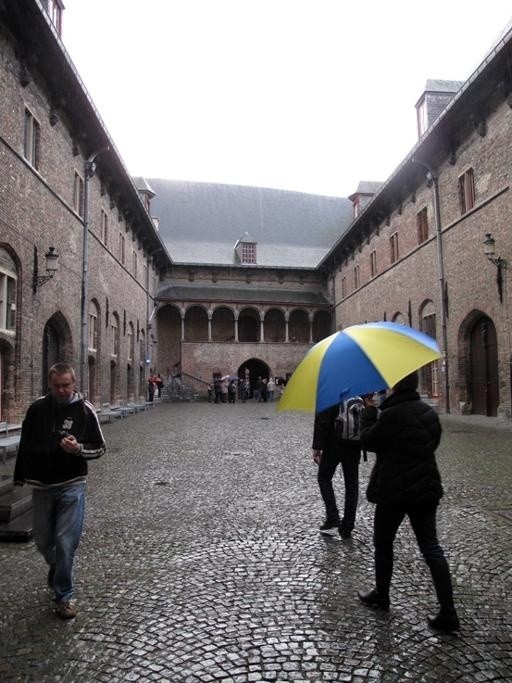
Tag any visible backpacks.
[334,395,367,441]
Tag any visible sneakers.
[320,515,343,530]
[60,596,76,620]
[337,527,352,538]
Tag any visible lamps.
[36,246,59,288]
[483,233,502,267]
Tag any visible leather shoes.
[358,589,390,610]
[427,608,460,631]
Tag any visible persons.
[208,371,289,405]
[313,396,367,537]
[11,361,106,620]
[147,373,156,405]
[155,373,163,397]
[360,366,460,631]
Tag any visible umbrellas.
[273,322,446,414]
